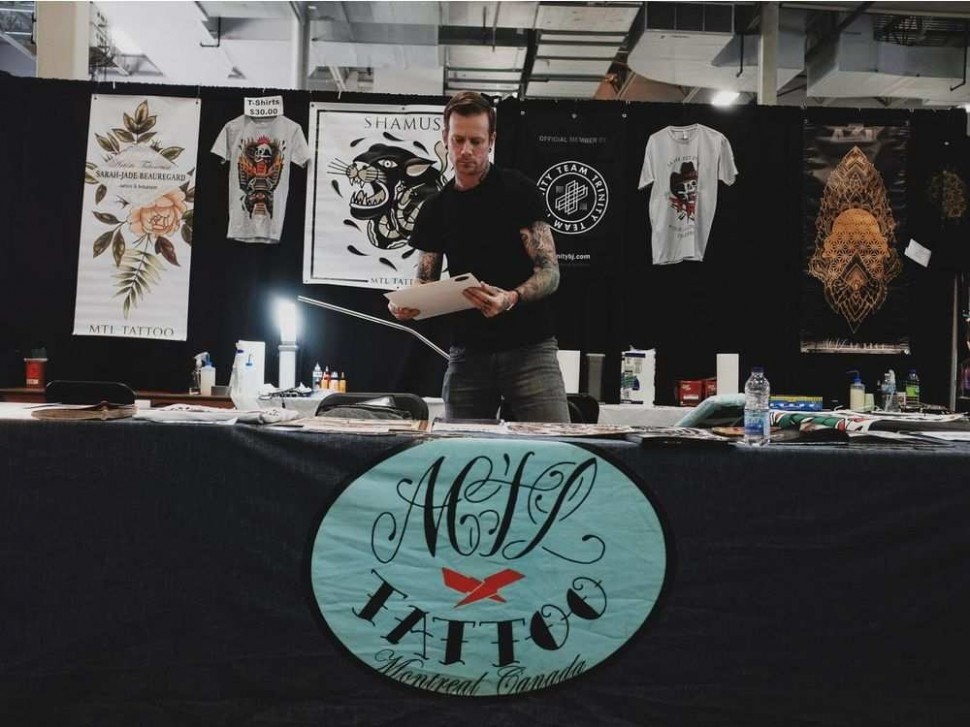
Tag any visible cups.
[23,358,48,388]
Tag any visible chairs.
[45,379,138,407]
[499,394,599,424]
[315,391,429,422]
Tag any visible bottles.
[201,354,216,394]
[881,373,896,413]
[744,368,770,447]
[904,369,919,408]
[312,362,346,394]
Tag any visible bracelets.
[512,289,522,303]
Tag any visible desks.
[0,400,970,727]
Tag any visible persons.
[388,92,571,423]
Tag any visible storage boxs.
[673,378,717,408]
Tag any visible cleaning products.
[846,370,865,412]
[191,350,220,399]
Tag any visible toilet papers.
[716,353,740,395]
